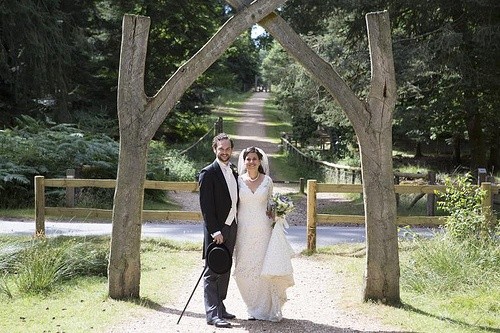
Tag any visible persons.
[232,146,295,322]
[199,134,241,328]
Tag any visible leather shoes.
[207,318,231,328]
[219,312,235,319]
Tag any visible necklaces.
[247,172,259,181]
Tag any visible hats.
[206,241,232,274]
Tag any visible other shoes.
[248,317,256,320]
